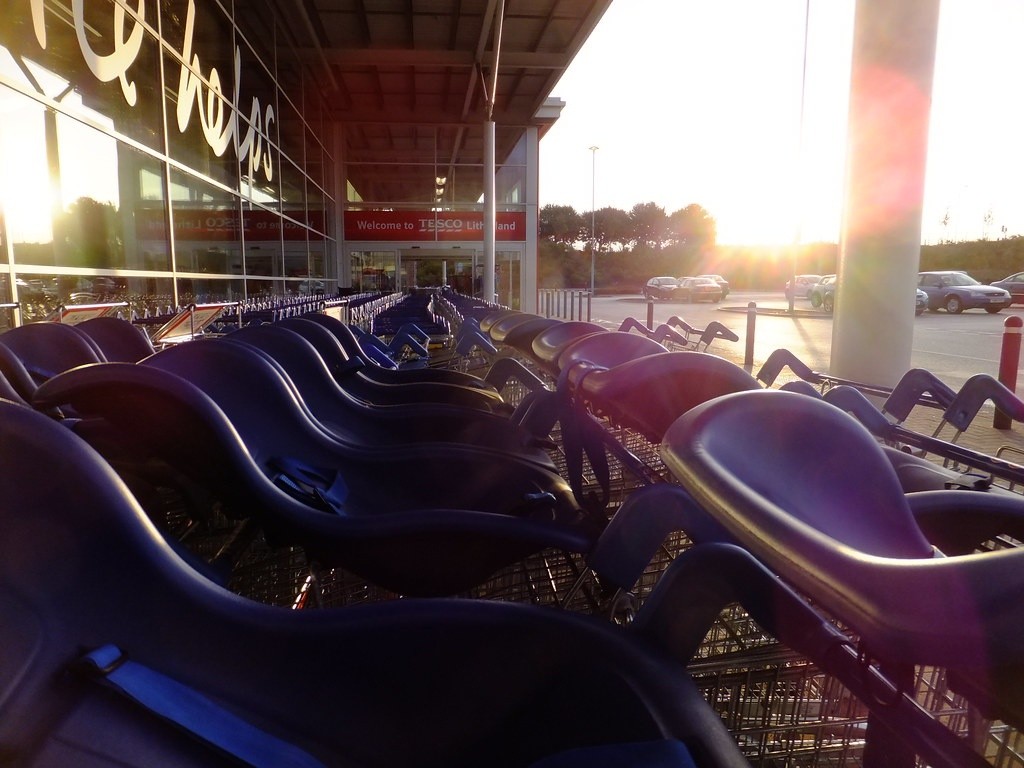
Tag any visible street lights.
[588,146,601,295]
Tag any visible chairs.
[0,284,1024,768]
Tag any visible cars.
[783,274,823,298]
[641,276,678,301]
[810,274,837,307]
[821,276,929,315]
[916,271,1012,315]
[670,276,723,303]
[990,271,1024,305]
[680,274,731,300]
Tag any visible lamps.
[433,175,446,203]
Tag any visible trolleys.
[0,283,1024,768]
